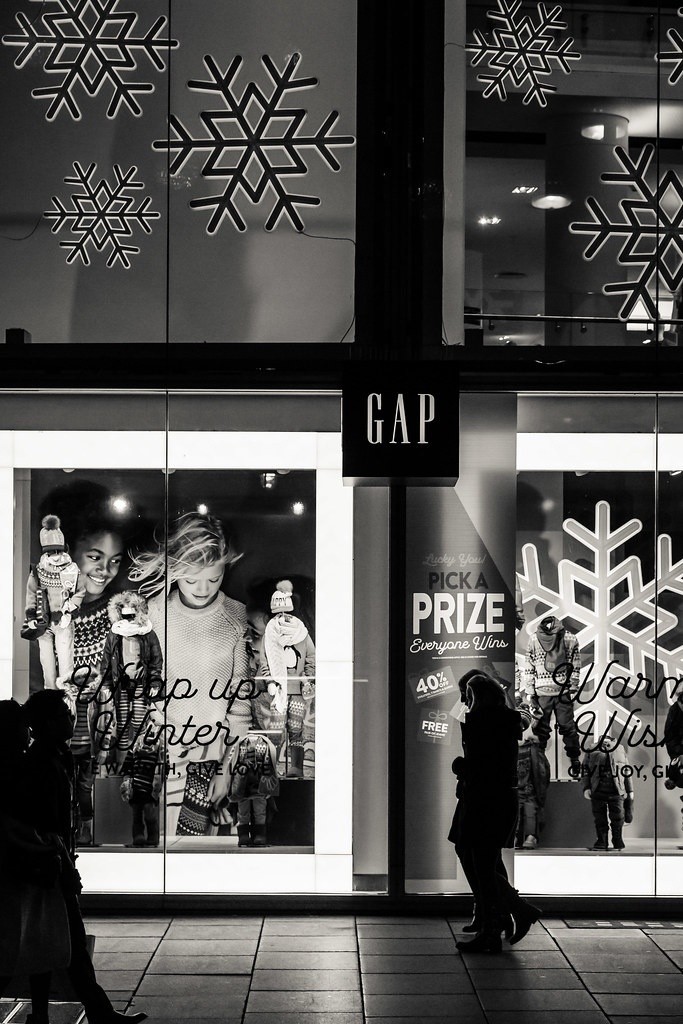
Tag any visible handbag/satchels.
[119,779,132,801]
[22,563,50,641]
[0,935,96,1001]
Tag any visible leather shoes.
[96,1009,148,1024]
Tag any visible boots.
[463,902,488,931]
[456,922,503,954]
[76,820,92,844]
[509,897,542,946]
[254,824,267,845]
[286,744,304,778]
[237,823,252,846]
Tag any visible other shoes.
[523,835,537,848]
[612,836,625,850]
[594,841,608,852]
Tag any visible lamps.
[261,471,278,488]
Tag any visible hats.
[40,515,64,552]
[270,581,293,613]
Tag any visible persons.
[242,574,315,779]
[101,591,163,763]
[25,515,86,690]
[123,721,170,845]
[48,479,146,668]
[581,735,634,850]
[447,669,542,952]
[132,512,252,836]
[260,580,315,776]
[515,702,547,848]
[525,615,583,779]
[0,689,148,1024]
[228,734,279,846]
